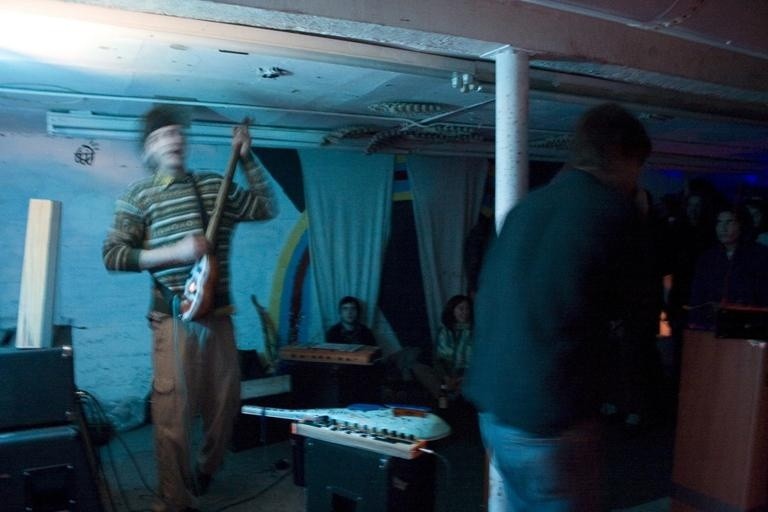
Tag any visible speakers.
[0,425,106,512]
[302,438,438,512]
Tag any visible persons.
[323,295,377,407]
[462,161,554,290]
[458,101,664,512]
[411,294,476,400]
[103,105,280,512]
[629,184,767,343]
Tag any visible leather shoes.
[190,466,211,494]
